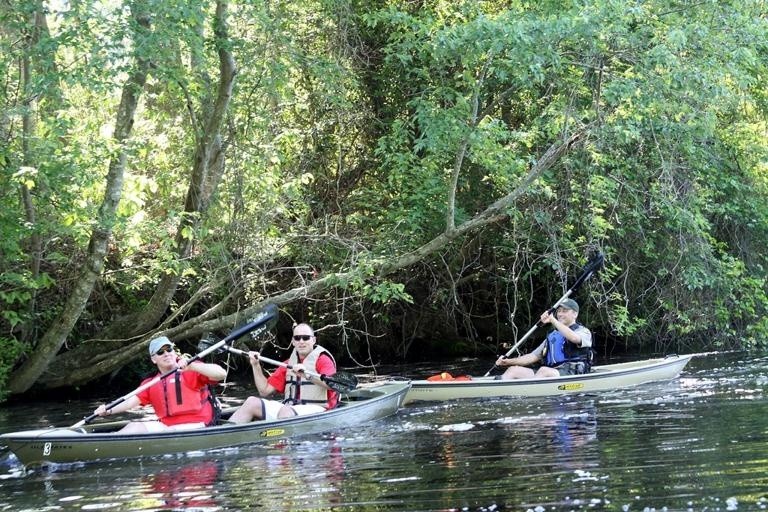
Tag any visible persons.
[496,298,593,380]
[223,324,342,427]
[93,336,228,435]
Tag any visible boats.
[403,354,695,405]
[0,378,413,472]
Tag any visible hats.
[149,336,175,356]
[559,299,580,314]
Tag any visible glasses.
[292,335,315,341]
[156,347,172,355]
